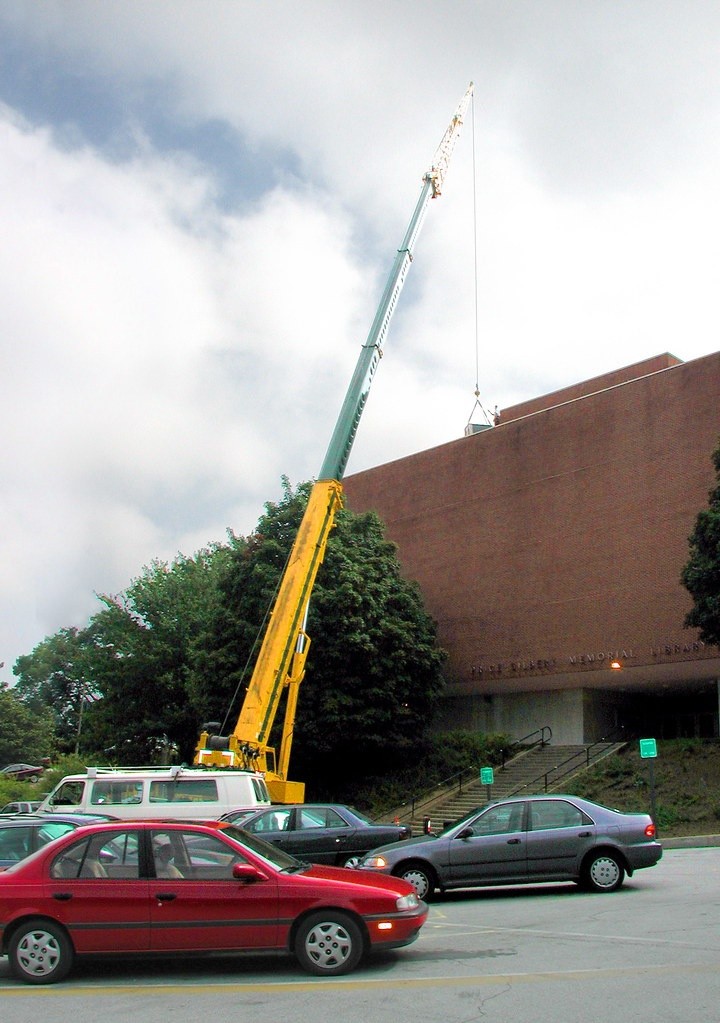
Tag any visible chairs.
[54,842,109,878]
[159,844,185,878]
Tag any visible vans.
[33,766,274,844]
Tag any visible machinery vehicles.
[106,79,480,805]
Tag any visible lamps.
[610,662,621,669]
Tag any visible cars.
[160,803,414,866]
[0,763,45,783]
[0,818,430,985]
[0,801,218,872]
[355,794,665,903]
